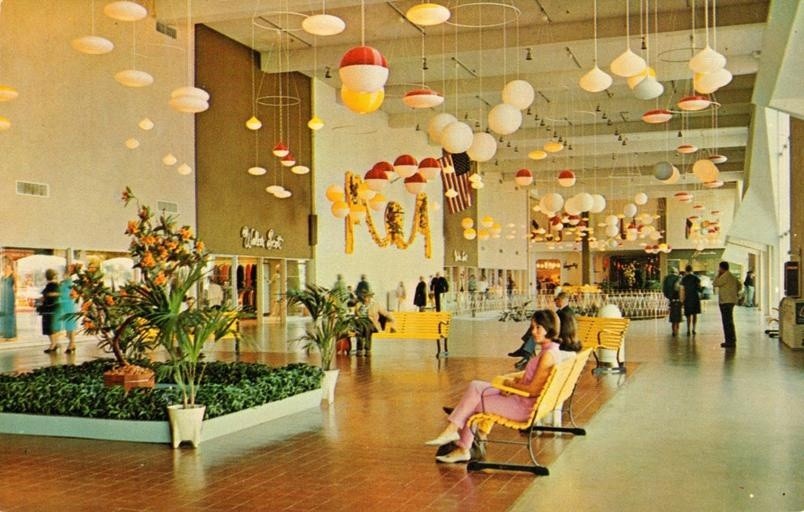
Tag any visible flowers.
[60,182,208,374]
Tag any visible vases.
[98,363,157,397]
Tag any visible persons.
[38,269,62,353]
[662,262,756,347]
[59,271,79,352]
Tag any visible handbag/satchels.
[679,284,686,303]
[435,416,488,463]
[744,281,748,287]
[559,342,582,352]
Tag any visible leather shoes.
[435,447,473,462]
[721,340,736,348]
[424,431,461,446]
[507,348,530,359]
[515,358,528,368]
[442,406,455,415]
[687,330,695,336]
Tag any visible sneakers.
[356,349,370,357]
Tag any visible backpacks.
[663,274,679,299]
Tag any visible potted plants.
[106,242,255,453]
[285,310,381,404]
[276,278,359,356]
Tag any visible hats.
[670,267,678,273]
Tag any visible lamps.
[580,1,731,242]
[325,171,387,222]
[246,0,345,199]
[468,173,485,189]
[445,189,458,198]
[177,163,192,176]
[645,245,659,254]
[444,166,455,174]
[0,116,11,130]
[0,84,18,103]
[505,223,526,240]
[364,0,534,190]
[170,2,210,114]
[162,153,177,166]
[529,215,660,247]
[338,1,389,114]
[72,2,154,87]
[125,137,140,149]
[515,21,605,215]
[461,209,502,241]
[606,136,648,218]
[139,118,154,131]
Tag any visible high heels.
[64,347,76,353]
[44,344,58,353]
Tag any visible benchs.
[465,344,596,475]
[569,314,633,376]
[333,305,453,362]
